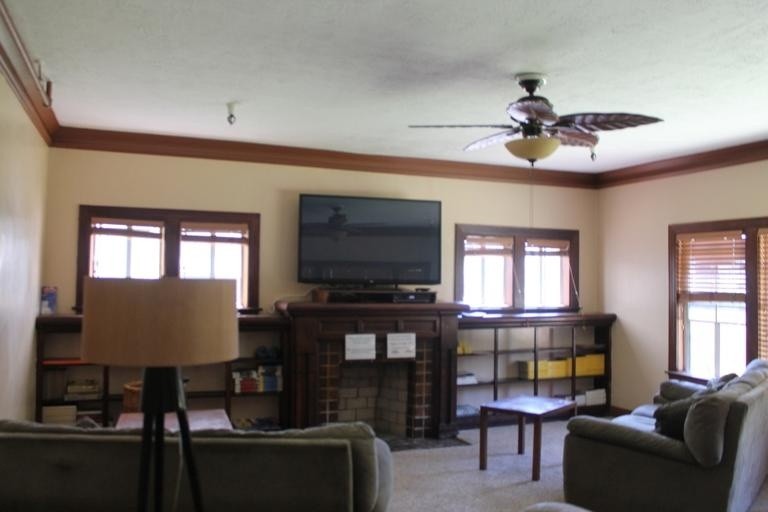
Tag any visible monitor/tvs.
[297,194,441,285]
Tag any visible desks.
[480,393,578,482]
[114,409,233,431]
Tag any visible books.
[231,364,284,393]
[42,377,104,429]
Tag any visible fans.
[407,74,663,151]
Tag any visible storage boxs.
[517,354,607,380]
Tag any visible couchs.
[563,358,767,511]
[0,416,391,512]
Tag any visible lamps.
[81,277,238,510]
[505,121,559,165]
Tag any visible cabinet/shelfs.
[224,318,294,431]
[457,313,616,431]
[35,317,109,427]
